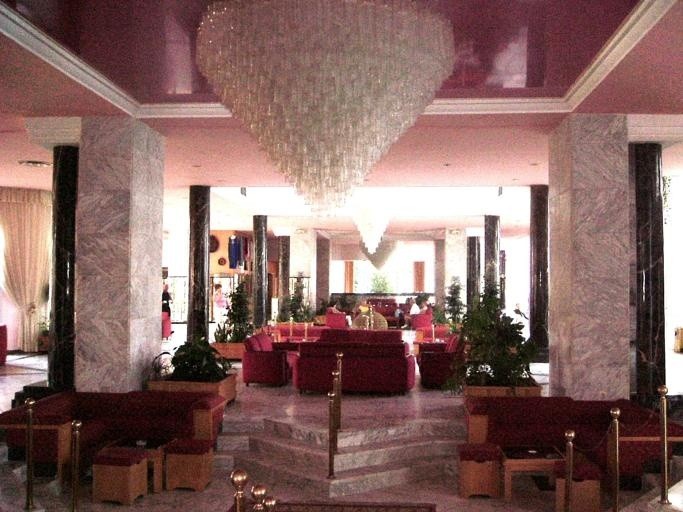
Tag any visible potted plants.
[147,334,238,405]
[440,284,542,414]
[211,281,254,359]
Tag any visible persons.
[326,300,349,327]
[160,283,172,340]
[400,301,428,330]
[214,283,227,320]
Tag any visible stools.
[92,448,148,505]
[458,443,500,498]
[164,439,213,492]
[553,461,601,512]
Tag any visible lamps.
[197,0,454,222]
[358,238,395,270]
[352,214,388,254]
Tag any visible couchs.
[464,395,683,488]
[243,300,464,394]
[0,390,226,490]
